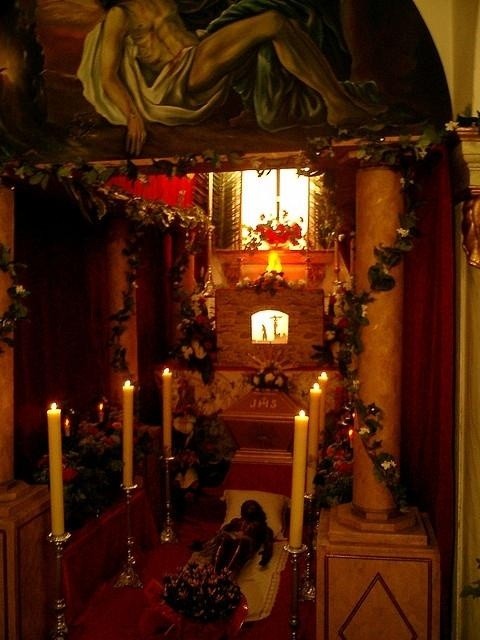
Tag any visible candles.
[47,373,170,538]
[290,376,327,549]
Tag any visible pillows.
[219,490,289,541]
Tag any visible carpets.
[196,535,289,622]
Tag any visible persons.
[190,499,274,579]
